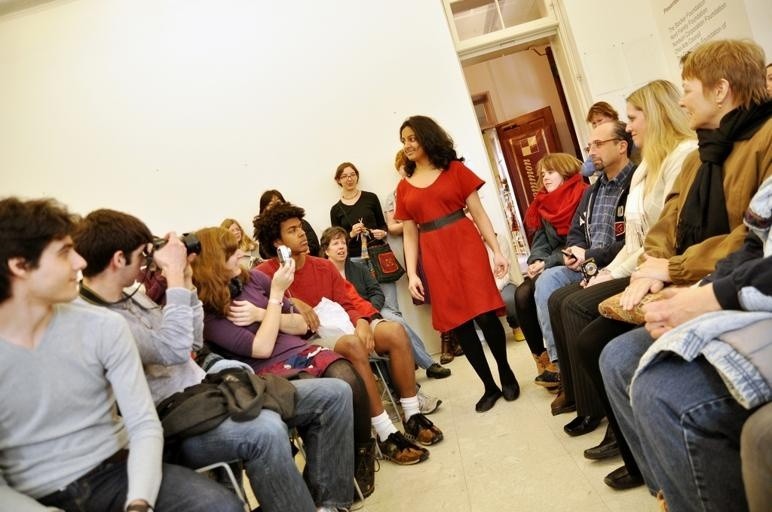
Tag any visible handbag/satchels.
[597,285,687,328]
[362,228,405,285]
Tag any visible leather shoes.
[551,381,575,416]
[583,424,620,460]
[602,465,645,490]
[512,325,528,342]
[472,384,501,413]
[564,412,605,436]
[453,340,465,356]
[439,348,454,364]
[501,372,521,402]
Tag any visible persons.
[508,39,771,510]
[1,162,453,510]
[386,117,521,412]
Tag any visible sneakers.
[376,429,430,467]
[426,361,452,379]
[416,385,442,415]
[402,412,443,446]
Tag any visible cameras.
[581,257,599,284]
[149,234,203,258]
[277,245,292,263]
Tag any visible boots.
[532,347,561,393]
[352,438,377,499]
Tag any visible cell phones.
[562,249,577,261]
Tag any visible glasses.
[340,173,357,180]
[586,136,623,151]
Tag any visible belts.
[418,207,466,233]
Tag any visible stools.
[293,427,364,511]
[195,458,250,512]
[370,360,401,421]
